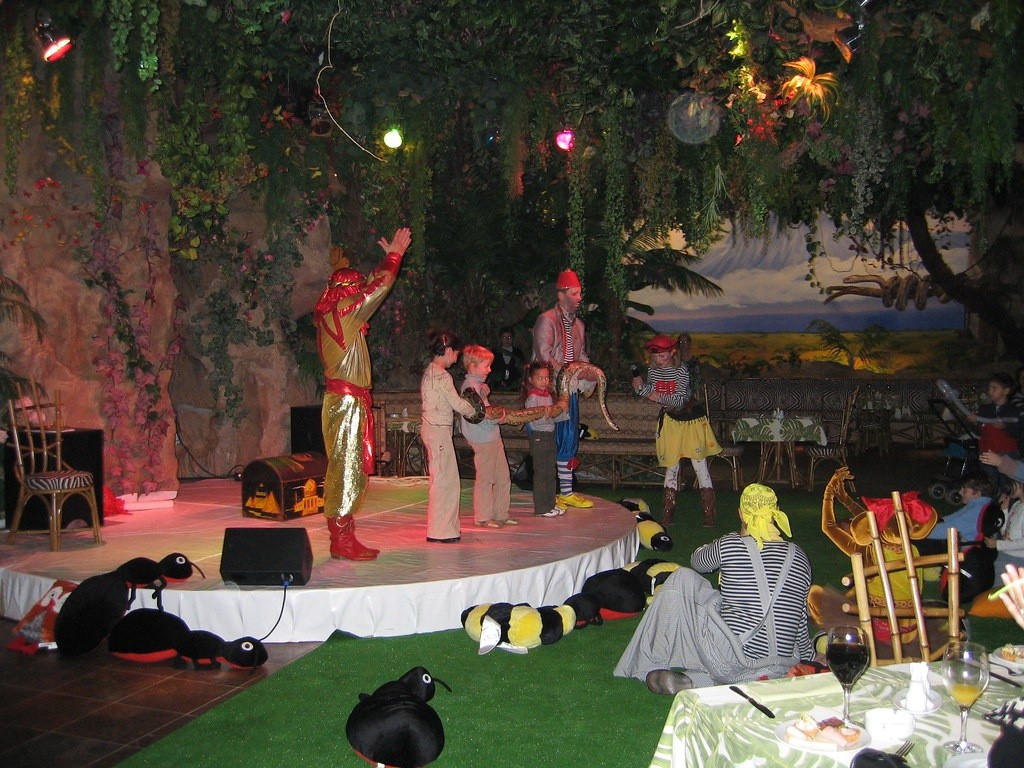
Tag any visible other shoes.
[536,509,559,518]
[553,506,565,515]
[427,536,460,544]
[646,669,693,696]
[503,516,517,525]
[475,521,499,529]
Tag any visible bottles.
[402,408,409,418]
[773,410,784,418]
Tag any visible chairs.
[849,490,968,670]
[693,382,744,490]
[806,386,862,495]
[7,375,102,554]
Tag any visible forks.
[895,740,915,758]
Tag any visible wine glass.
[943,642,989,753]
[826,626,870,729]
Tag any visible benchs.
[372,390,687,491]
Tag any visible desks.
[731,414,828,489]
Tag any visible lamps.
[34,18,73,63]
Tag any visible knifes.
[729,685,775,720]
[989,670,1021,688]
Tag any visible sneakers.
[558,494,594,507]
[554,502,567,510]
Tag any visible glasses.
[502,336,512,339]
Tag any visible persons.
[313,226,411,559]
[525,363,567,517]
[420,332,465,543]
[632,333,723,528]
[532,269,597,508]
[613,483,827,694]
[927,367,1024,630]
[491,327,525,388]
[461,344,522,529]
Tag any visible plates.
[943,752,988,768]
[774,721,871,754]
[892,688,942,715]
[993,645,1024,666]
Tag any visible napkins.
[905,662,934,711]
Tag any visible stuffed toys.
[940,488,1011,606]
[56,552,292,669]
[346,665,453,768]
[619,497,674,553]
[808,466,948,660]
[461,557,685,654]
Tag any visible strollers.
[926,398,981,508]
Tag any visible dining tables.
[649,656,1024,768]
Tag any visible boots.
[661,486,675,529]
[327,514,377,561]
[699,487,715,528]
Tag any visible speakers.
[220,528,314,586]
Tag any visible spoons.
[987,660,1022,676]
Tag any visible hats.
[645,335,678,354]
[557,268,581,290]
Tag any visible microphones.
[630,365,642,392]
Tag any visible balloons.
[936,379,1018,423]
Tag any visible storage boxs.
[241,451,328,522]
